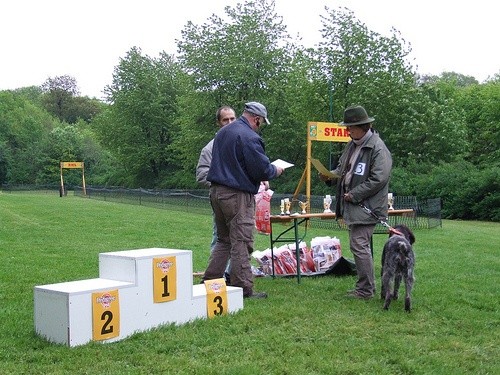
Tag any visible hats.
[337,105,375,126]
[245,102,270,125]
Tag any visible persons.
[201,102,283,299]
[196,107,237,278]
[318,105,393,299]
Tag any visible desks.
[269,208,413,283]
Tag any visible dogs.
[380,225,416,312]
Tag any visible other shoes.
[345,294,374,300]
[243,291,267,298]
[346,289,375,296]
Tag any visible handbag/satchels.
[254,181,274,236]
[252,236,341,274]
